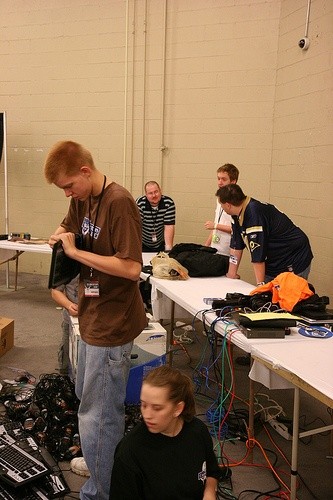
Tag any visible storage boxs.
[0,316,14,357]
[68,313,166,405]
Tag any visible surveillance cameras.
[298,37,310,50]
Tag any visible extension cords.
[176,320,194,331]
[267,414,296,439]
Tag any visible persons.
[215,184,314,297]
[108,364,221,500]
[204,163,239,273]
[135,180,176,317]
[42,139,149,500]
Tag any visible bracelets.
[214,223,217,229]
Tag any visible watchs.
[256,281,266,288]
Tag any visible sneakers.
[70,457,90,477]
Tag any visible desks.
[0,238,53,291]
[140,253,333,500]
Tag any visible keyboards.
[0,421,70,500]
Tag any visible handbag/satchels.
[48,233,83,289]
[150,251,189,280]
[168,242,229,277]
